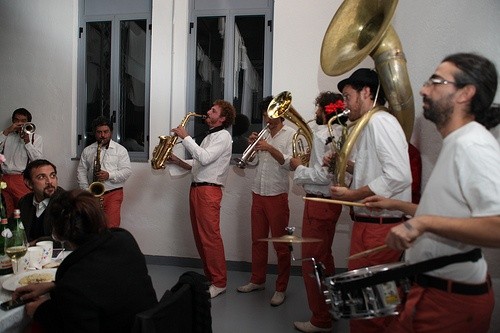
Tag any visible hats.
[338,68,387,104]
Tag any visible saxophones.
[88,140,108,199]
[150,109,208,170]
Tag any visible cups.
[11,241,53,274]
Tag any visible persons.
[8,159,67,249]
[13,188,158,333]
[363,53,500,333]
[322,68,413,333]
[236,96,297,305]
[76,117,131,228]
[0,107,43,217]
[166,100,236,297]
[289,91,356,333]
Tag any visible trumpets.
[17,123,36,138]
[233,121,273,170]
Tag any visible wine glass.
[4,230,27,274]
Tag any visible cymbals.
[256,233,327,246]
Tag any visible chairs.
[127,271,213,333]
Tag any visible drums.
[320,261,413,322]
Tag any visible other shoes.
[271,291,285,306]
[209,285,226,298]
[293,321,332,333]
[237,282,265,293]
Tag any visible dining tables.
[0,240,71,333]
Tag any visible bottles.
[0,191,28,275]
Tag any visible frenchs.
[319,0,415,189]
[266,89,315,167]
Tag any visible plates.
[2,268,57,291]
[43,258,64,268]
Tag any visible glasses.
[430,75,461,84]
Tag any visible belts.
[104,186,123,195]
[191,182,220,187]
[355,215,404,223]
[414,275,492,295]
